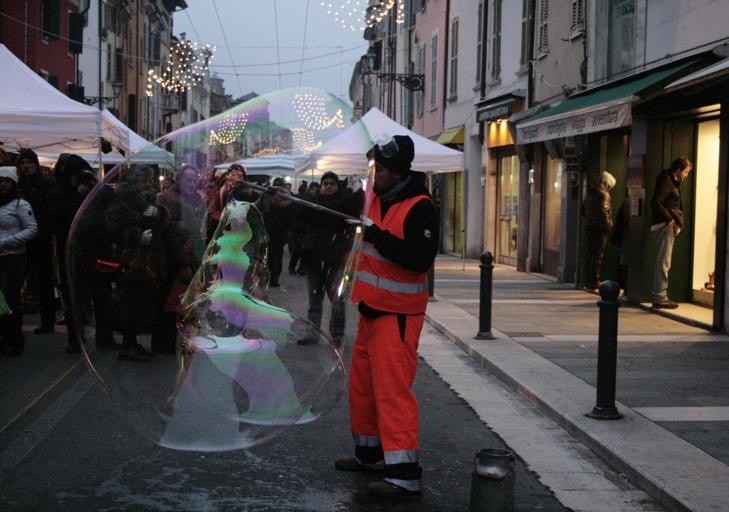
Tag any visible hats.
[0,166,19,185]
[366,135,415,174]
[321,171,339,186]
[17,147,38,166]
[126,163,155,186]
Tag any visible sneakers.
[368,480,423,498]
[297,332,321,346]
[33,323,56,334]
[653,300,678,309]
[335,455,377,473]
[66,341,84,354]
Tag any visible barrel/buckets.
[471,448,514,512]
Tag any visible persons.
[581,169,618,294]
[609,197,628,298]
[331,135,442,503]
[2,148,208,361]
[647,155,694,311]
[203,149,365,346]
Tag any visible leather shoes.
[118,344,155,363]
